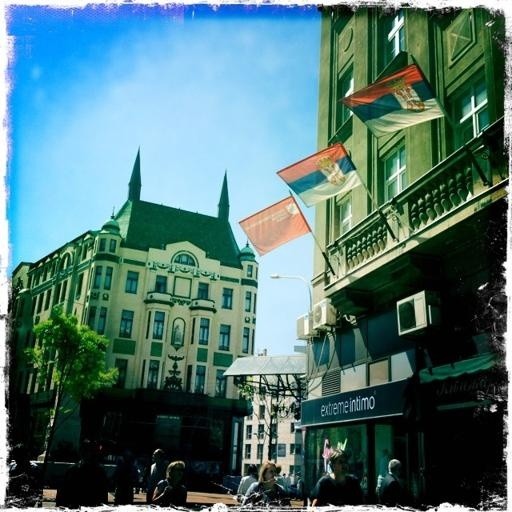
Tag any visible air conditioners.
[295,289,443,340]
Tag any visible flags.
[274,139,361,212]
[335,63,445,139]
[237,196,311,261]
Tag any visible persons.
[108,448,187,507]
[8,443,41,506]
[54,440,108,506]
[238,461,303,511]
[378,459,406,505]
[310,449,364,508]
